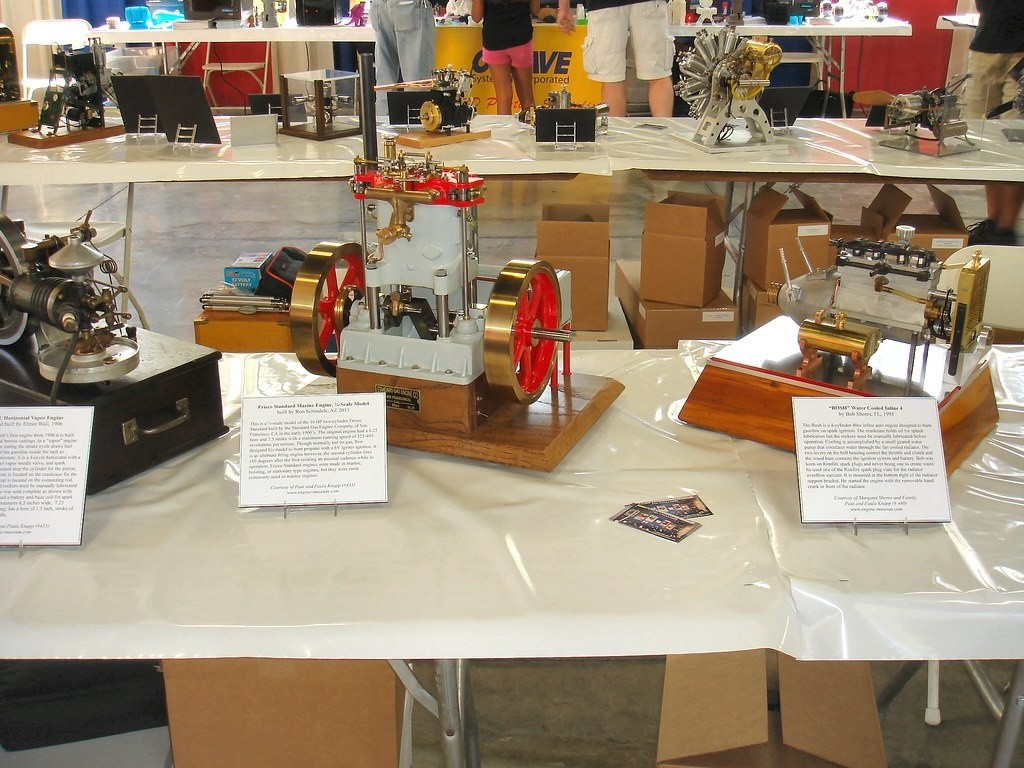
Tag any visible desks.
[91,16,377,93]
[668,12,914,112]
[2,317,1024,768]
[430,9,608,112]
[0,113,1024,329]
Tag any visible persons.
[555,0,678,117]
[369,0,437,117]
[470,0,543,114]
[958,1,1024,235]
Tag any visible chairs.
[201,41,271,108]
[19,19,96,99]
